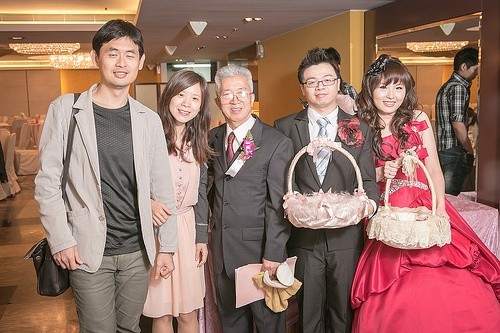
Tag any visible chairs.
[420,103,435,127]
[0,112,47,197]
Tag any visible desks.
[196,193,500,333]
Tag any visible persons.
[273,50,379,333]
[349,54,500,333]
[141,69,218,333]
[323,46,359,102]
[205,64,296,333]
[436,47,479,198]
[35,18,178,333]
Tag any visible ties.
[226,133,235,162]
[313,117,331,182]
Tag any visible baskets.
[282,139,368,228]
[366,153,452,249]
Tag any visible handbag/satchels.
[24,236,71,297]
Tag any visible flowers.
[338,117,364,149]
[239,130,261,162]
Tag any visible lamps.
[8,42,80,56]
[165,46,177,56]
[407,42,471,52]
[187,20,207,38]
[49,53,98,69]
[440,22,455,37]
[147,64,156,70]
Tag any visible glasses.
[302,76,339,88]
[220,90,251,104]
